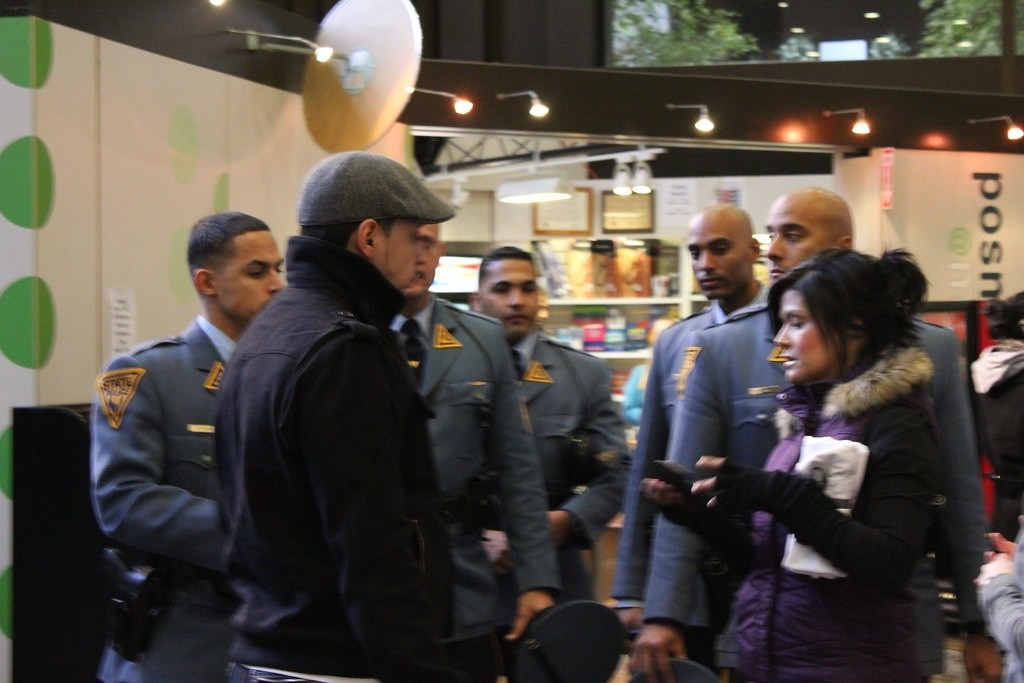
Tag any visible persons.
[621,316,676,424]
[972,511,1024,683]
[214,151,458,683]
[391,223,562,683]
[89,210,287,683]
[971,292,1024,542]
[627,187,1002,683]
[641,247,948,683]
[470,246,631,630]
[610,202,764,657]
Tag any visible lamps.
[495,149,572,203]
[450,175,471,209]
[613,152,653,196]
[414,89,1024,139]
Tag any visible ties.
[399,319,427,383]
[511,349,524,381]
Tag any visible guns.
[102,546,158,664]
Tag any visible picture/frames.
[533,187,594,236]
[602,189,654,234]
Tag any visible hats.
[507,600,623,683]
[296,151,457,225]
[628,659,722,683]
